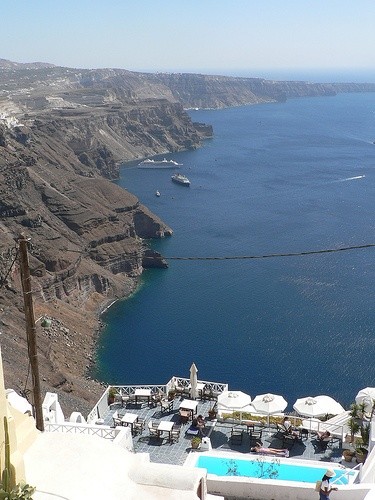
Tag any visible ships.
[136,157,180,169]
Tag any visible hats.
[324,469,336,478]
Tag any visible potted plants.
[109,387,116,404]
[355,437,368,464]
[343,448,354,462]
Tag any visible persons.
[192,414,208,437]
[283,417,299,439]
[251,442,283,455]
[311,430,331,442]
[319,469,338,500]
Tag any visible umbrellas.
[252,393,288,426]
[292,397,327,436]
[355,387,375,414]
[217,391,252,432]
[190,362,199,398]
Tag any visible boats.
[171,173,190,185]
[156,190,160,196]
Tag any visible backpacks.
[315,480,322,492]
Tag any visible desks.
[112,383,341,449]
